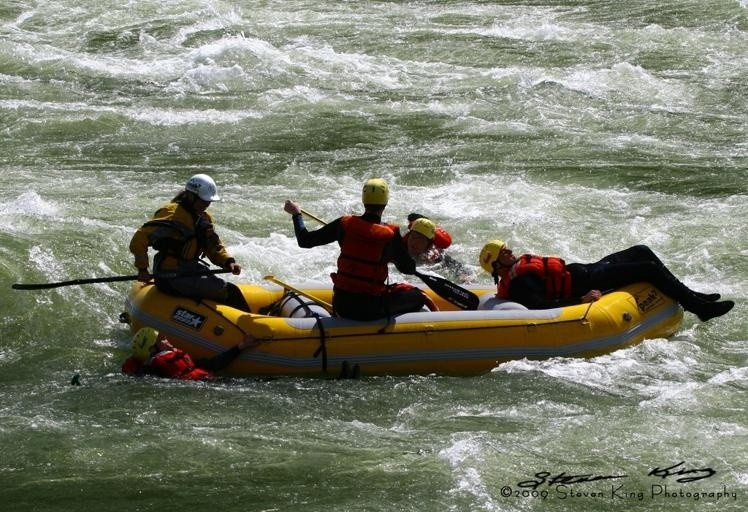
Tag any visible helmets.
[131,327,159,361]
[478,240,505,273]
[409,218,435,240]
[185,174,219,201]
[362,179,389,205]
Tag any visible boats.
[119,279,686,375]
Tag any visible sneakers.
[695,292,733,322]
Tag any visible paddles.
[13,269,231,289]
[299,208,480,313]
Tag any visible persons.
[284,178,425,323]
[121,327,260,385]
[479,240,735,323]
[129,174,252,314]
[401,213,463,274]
